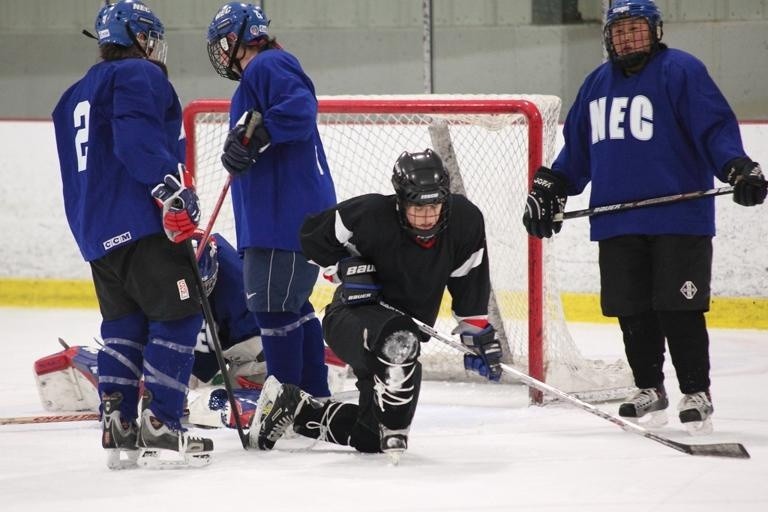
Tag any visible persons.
[206,0,338,402]
[521,0,767,433]
[63,227,268,428]
[246,148,502,464]
[52,0,216,468]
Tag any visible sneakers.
[619,388,668,417]
[378,422,409,450]
[679,391,713,423]
[257,383,310,450]
[138,407,214,454]
[102,410,139,450]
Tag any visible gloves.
[221,111,270,176]
[461,324,503,383]
[338,256,381,307]
[722,156,767,206]
[151,163,201,245]
[523,166,567,239]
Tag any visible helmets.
[190,227,220,304]
[207,3,269,81]
[603,0,663,75]
[95,0,168,64]
[391,148,450,239]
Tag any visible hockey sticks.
[185,238,250,451]
[379,301,750,459]
